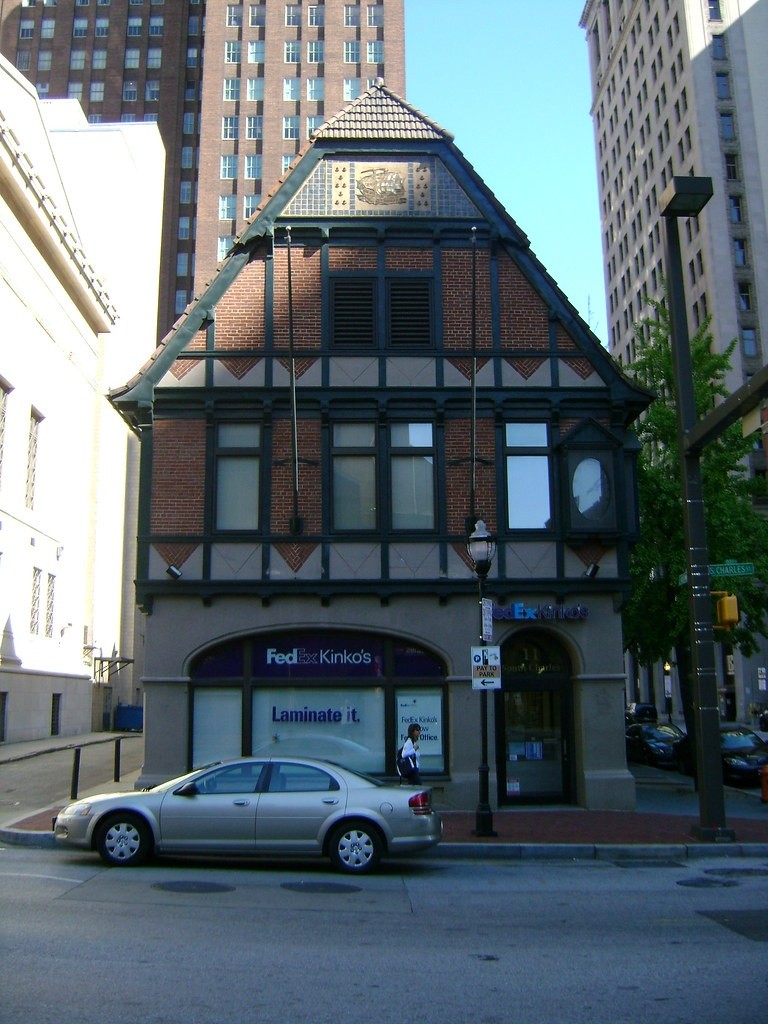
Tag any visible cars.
[671,723,768,788]
[230,733,381,774]
[626,702,671,724]
[52,752,442,875]
[625,721,688,769]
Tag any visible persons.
[400,723,422,783]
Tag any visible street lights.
[656,173,738,845]
[465,519,499,839]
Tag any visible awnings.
[95,656,135,675]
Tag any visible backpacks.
[395,736,410,782]
